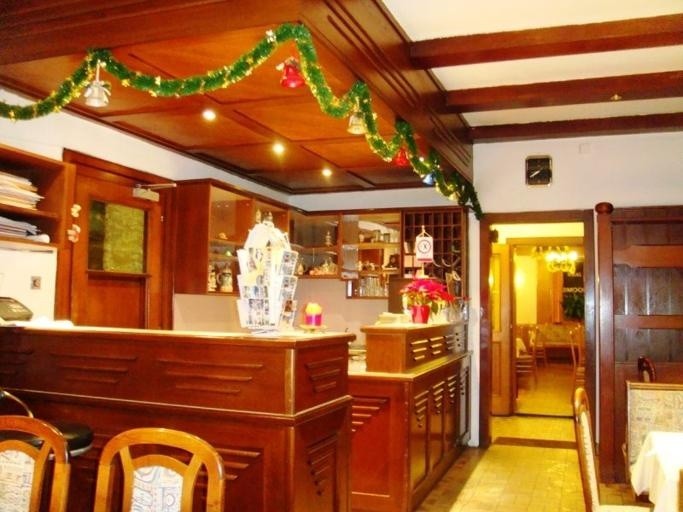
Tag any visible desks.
[645,432,683,512]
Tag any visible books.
[0,162,50,246]
[234,219,300,334]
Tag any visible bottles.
[325,229,332,246]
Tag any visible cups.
[208,264,233,292]
[359,230,390,243]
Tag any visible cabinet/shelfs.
[0,144,77,252]
[174,177,470,300]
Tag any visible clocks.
[525,155,553,189]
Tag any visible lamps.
[545,245,577,273]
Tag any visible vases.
[410,303,431,323]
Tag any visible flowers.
[400,279,454,305]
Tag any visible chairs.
[0,415,227,512]
[572,387,651,512]
[515,323,586,391]
